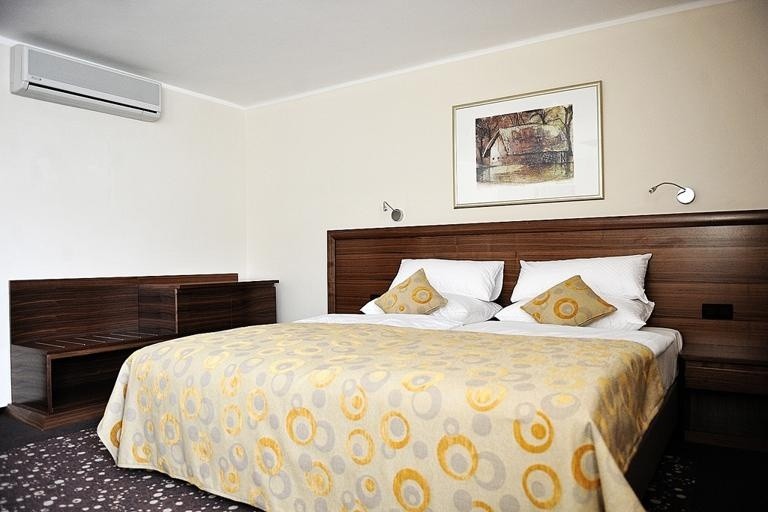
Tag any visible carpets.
[0,428,697,511]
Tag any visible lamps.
[382,200,405,223]
[648,181,696,204]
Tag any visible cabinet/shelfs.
[6,273,279,431]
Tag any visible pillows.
[360,252,656,332]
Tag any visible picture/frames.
[450,79,605,208]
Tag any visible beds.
[98,311,683,512]
